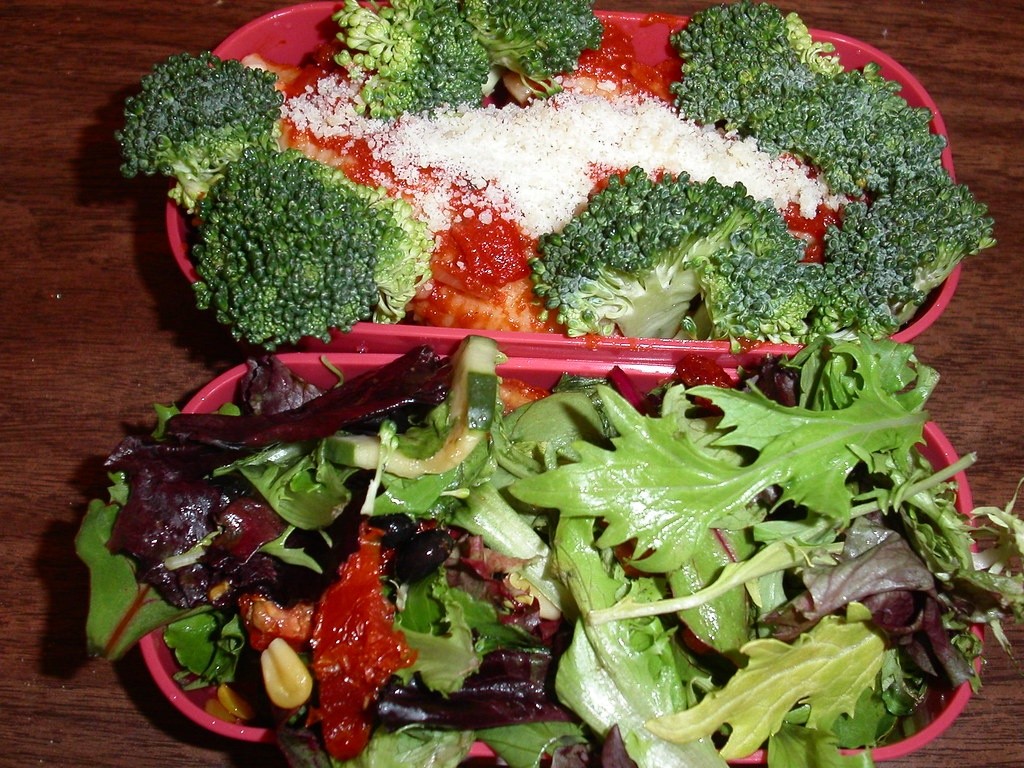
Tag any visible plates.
[166,0,963,365]
[136,353,984,761]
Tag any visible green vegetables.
[72,335,1024,768]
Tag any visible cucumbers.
[324,334,500,480]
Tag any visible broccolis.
[116,0,997,352]
